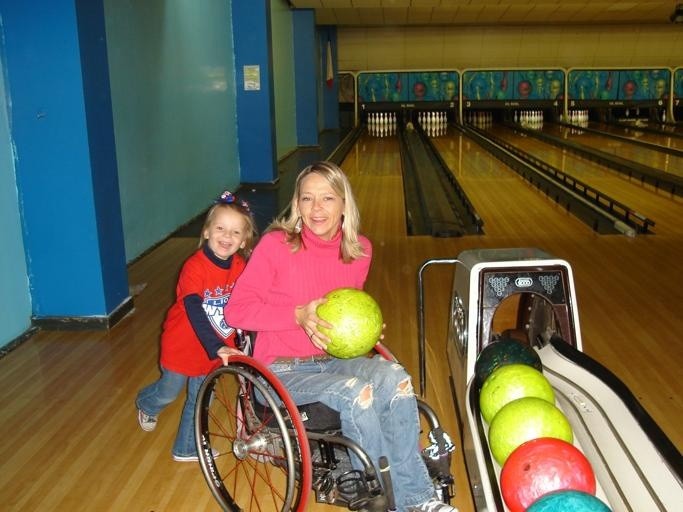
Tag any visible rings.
[311,333,314,338]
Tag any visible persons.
[223,161,458,512]
[136,190,259,462]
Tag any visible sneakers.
[406,497,459,512]
[174,449,219,462]
[139,409,158,432]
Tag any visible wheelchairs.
[194,329,455,512]
